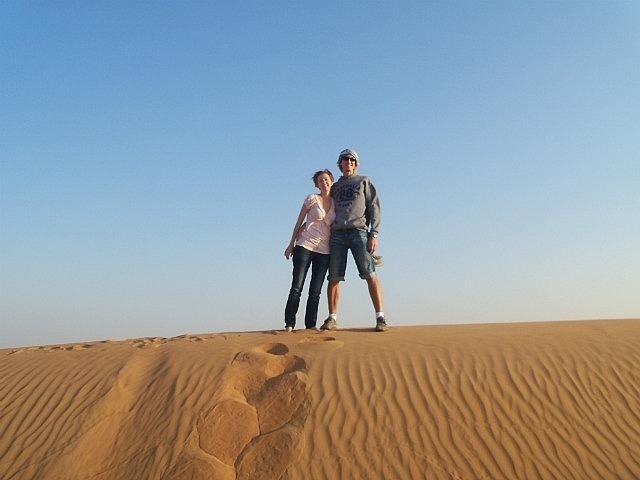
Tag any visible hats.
[338,149,358,172]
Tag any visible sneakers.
[376,316,387,331]
[320,317,336,330]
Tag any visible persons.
[285,169,338,333]
[295,147,390,331]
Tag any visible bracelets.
[369,234,380,237]
